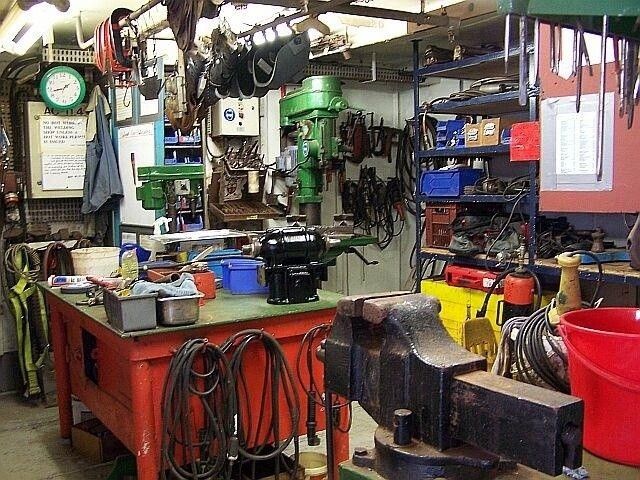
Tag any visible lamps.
[0,1,72,56]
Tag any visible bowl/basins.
[70,246,121,278]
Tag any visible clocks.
[39,65,85,108]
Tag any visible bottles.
[120,244,139,280]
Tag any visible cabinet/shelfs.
[529,19,640,278]
[410,10,538,272]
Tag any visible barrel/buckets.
[70,246,121,277]
[556,306,640,469]
[289,449,327,479]
